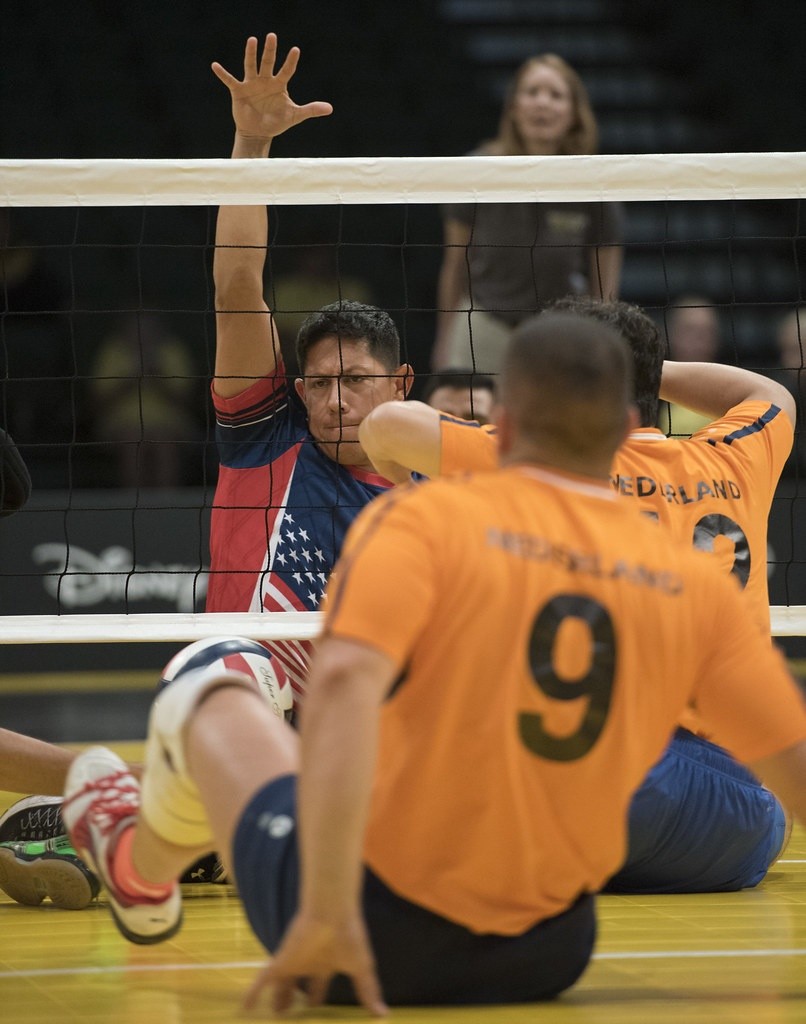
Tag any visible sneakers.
[0,834,101,910]
[62,744,185,946]
[0,795,67,841]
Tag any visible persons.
[211,33,806,734]
[0,240,806,1017]
